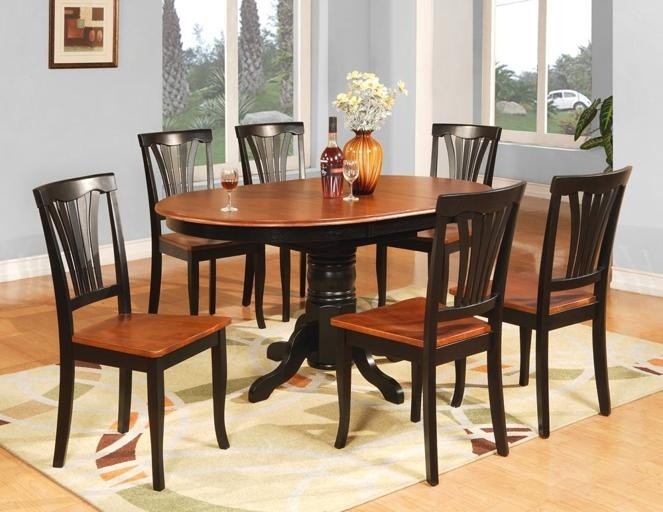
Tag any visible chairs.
[330,181,528,486]
[235,122,306,322]
[449,165,633,439]
[137,128,267,329]
[33,172,230,491]
[376,123,502,306]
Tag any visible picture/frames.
[49,0,119,69]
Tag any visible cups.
[342,159,360,201]
[220,167,239,212]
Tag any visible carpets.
[1,281,663,512]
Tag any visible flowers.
[332,71,408,131]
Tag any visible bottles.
[320,117,343,199]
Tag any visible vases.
[343,129,383,195]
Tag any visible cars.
[546,89,590,110]
